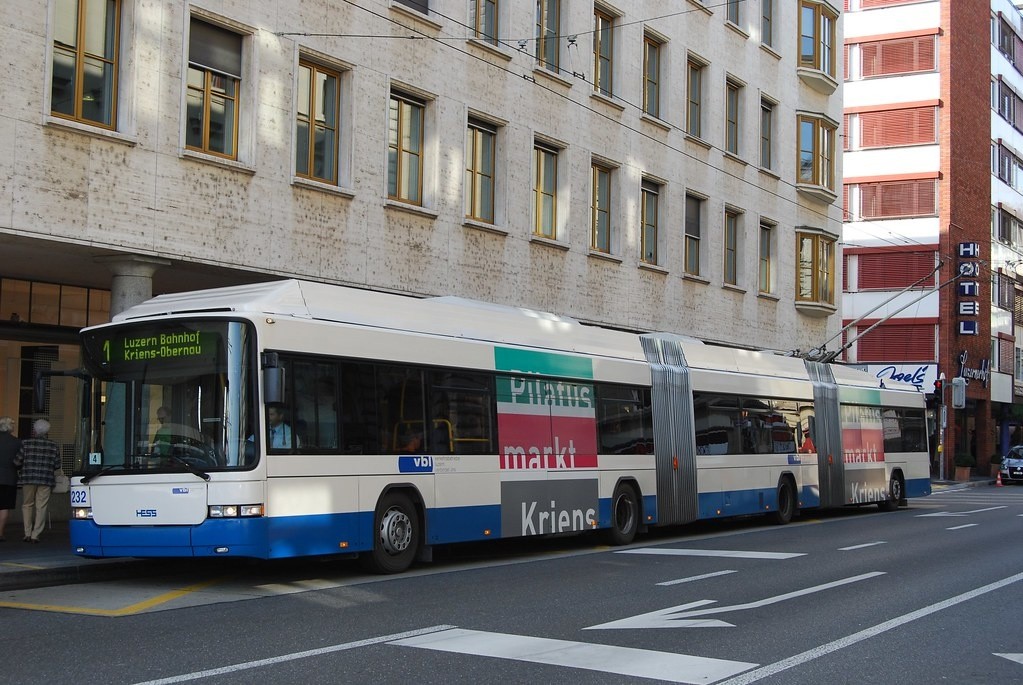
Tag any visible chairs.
[296,419,309,450]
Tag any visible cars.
[999,445,1023,484]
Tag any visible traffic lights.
[933,380,941,402]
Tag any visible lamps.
[1006,259,1023,268]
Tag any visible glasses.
[157,416,166,420]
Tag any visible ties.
[270,429,276,448]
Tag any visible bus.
[32,277,932,574]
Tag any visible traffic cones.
[995,470,1004,487]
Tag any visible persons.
[396,429,421,451]
[1010,425,1023,446]
[0,416,22,542]
[248,406,301,448]
[13,419,61,544]
[151,406,173,454]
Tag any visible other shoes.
[32,539,40,544]
[22,536,31,542]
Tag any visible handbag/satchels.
[50,476,71,494]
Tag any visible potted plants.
[953,452,975,482]
[990,453,1002,479]
